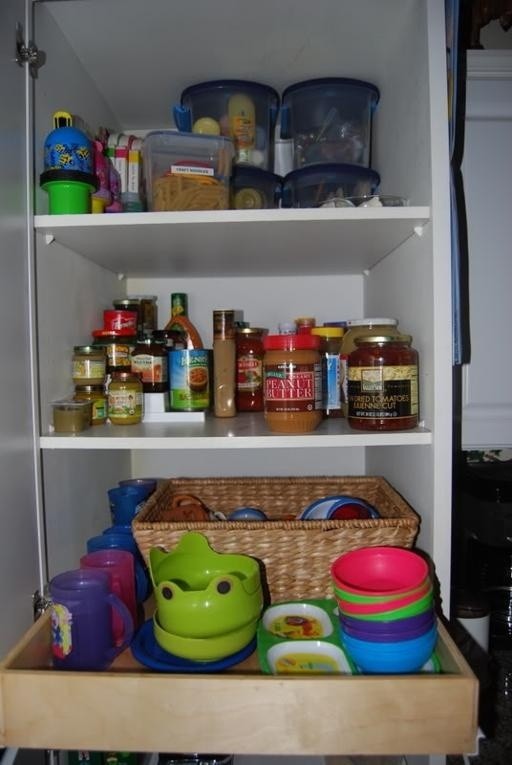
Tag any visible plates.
[128,619,256,672]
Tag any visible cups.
[47,569,133,671]
[120,479,158,496]
[86,536,145,607]
[107,486,149,523]
[80,549,137,646]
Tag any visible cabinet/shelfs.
[458,47,511,454]
[1,1,483,764]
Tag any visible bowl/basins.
[152,534,258,662]
[329,547,439,675]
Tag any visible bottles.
[311,327,345,417]
[164,292,205,350]
[263,335,324,433]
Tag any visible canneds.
[238,317,419,432]
[72,294,181,426]
[168,349,211,413]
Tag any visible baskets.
[132,476,420,606]
